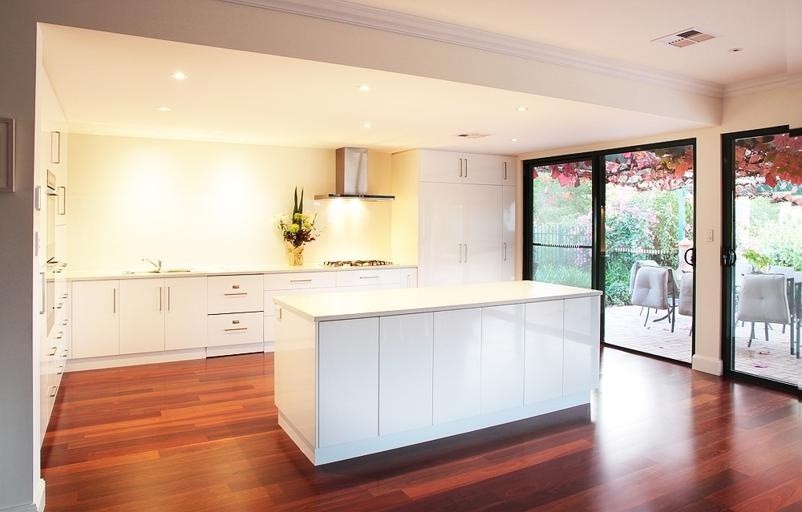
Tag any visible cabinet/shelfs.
[207,273,267,360]
[390,151,504,186]
[263,271,319,355]
[317,267,419,290]
[504,187,518,280]
[390,180,504,280]
[31,61,72,448]
[63,279,120,373]
[273,278,605,467]
[504,154,519,187]
[120,275,207,370]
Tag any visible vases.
[283,240,305,266]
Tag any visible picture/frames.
[1,117,17,196]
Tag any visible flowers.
[278,185,323,245]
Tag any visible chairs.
[629,255,802,362]
